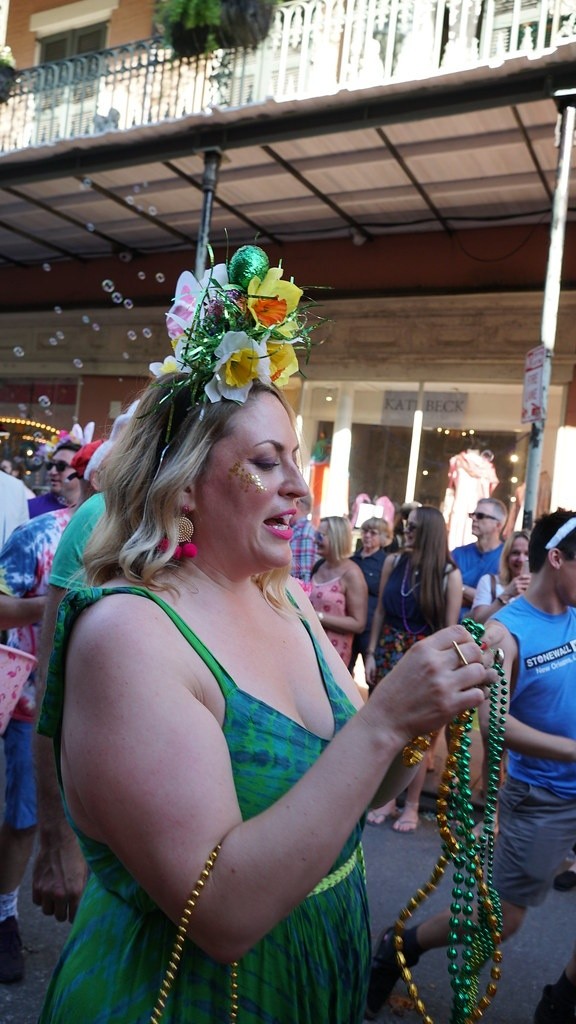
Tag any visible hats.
[69,439,109,480]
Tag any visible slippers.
[366,806,397,827]
[392,815,421,834]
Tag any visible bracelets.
[498,596,507,605]
[317,612,323,621]
[365,651,375,656]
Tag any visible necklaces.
[151,841,238,1024]
[401,560,420,597]
[392,619,507,1024]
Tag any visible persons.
[289,489,534,841]
[0,404,140,983]
[367,508,576,1024]
[37,374,497,1024]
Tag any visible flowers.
[136,244,336,443]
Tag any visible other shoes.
[363,927,420,1019]
[427,750,436,771]
[534,984,576,1024]
[553,870,576,892]
[471,820,498,849]
[0,916,27,987]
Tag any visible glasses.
[313,530,327,542]
[43,457,73,473]
[403,520,417,532]
[468,512,500,522]
[362,528,379,537]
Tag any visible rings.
[453,641,468,666]
